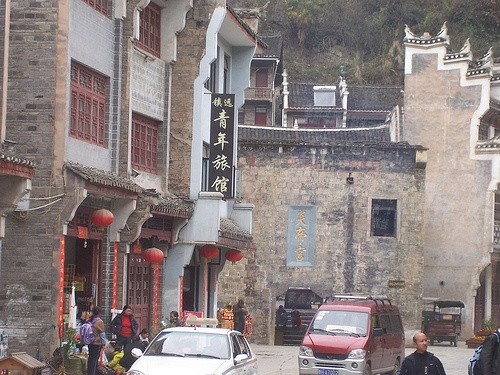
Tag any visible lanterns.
[225,249,243,265]
[201,244,219,262]
[91,208,113,231]
[143,247,165,270]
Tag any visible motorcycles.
[422,299,465,347]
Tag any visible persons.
[291,306,302,329]
[400,332,447,375]
[165,306,202,327]
[479,328,500,375]
[233,299,248,337]
[87,306,106,375]
[108,305,156,375]
[224,304,234,320]
[276,305,288,328]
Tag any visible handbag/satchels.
[97,364,115,375]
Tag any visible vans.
[298,292,405,375]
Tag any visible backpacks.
[468,330,500,375]
[80,317,101,345]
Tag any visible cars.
[125,326,258,375]
